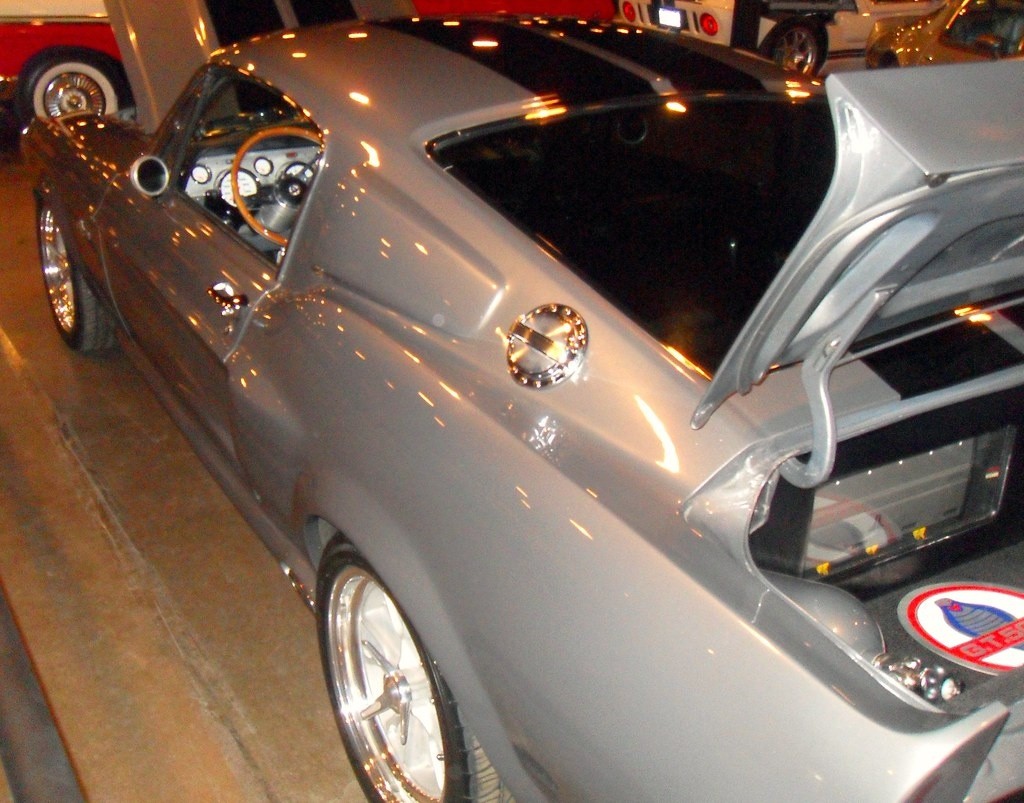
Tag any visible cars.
[21,16,1024,802]
[863,0,1024,71]
[0,1,616,130]
[617,0,948,76]
